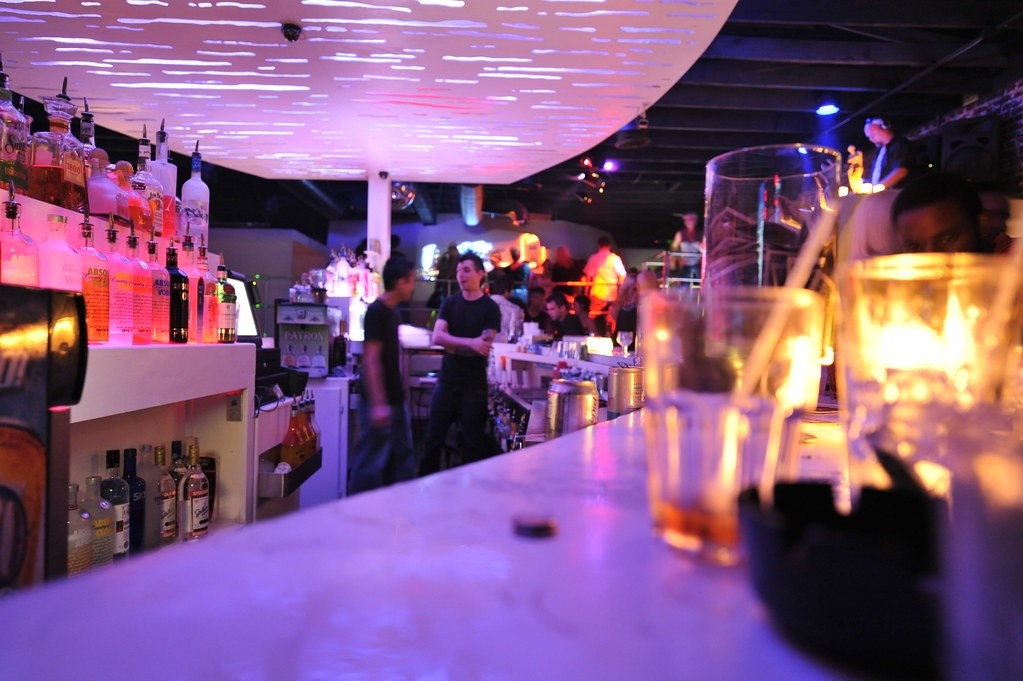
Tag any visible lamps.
[569,153,605,204]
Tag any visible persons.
[584,236,627,338]
[428,231,677,354]
[825,164,1023,257]
[417,249,503,478]
[856,113,919,189]
[347,254,418,494]
[672,212,702,287]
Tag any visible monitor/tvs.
[227,270,262,349]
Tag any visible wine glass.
[617,330,633,358]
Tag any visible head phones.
[865,117,891,129]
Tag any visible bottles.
[297,345,310,367]
[553,363,604,397]
[67,440,210,577]
[311,345,326,368]
[279,389,321,470]
[333,319,347,368]
[283,344,296,367]
[0,52,238,346]
[484,387,528,453]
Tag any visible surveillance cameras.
[280,21,304,42]
[379,171,389,179]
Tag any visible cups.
[698,143,840,507]
[347,267,380,298]
[313,287,326,305]
[557,340,577,360]
[638,283,826,569]
[835,186,1022,532]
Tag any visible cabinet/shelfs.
[68,344,359,576]
